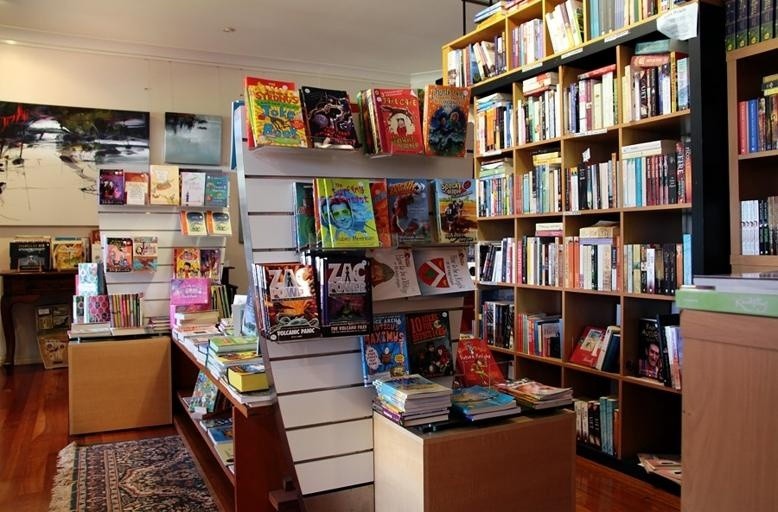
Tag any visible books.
[316,178,332,249]
[694,273,778,292]
[180,209,208,237]
[205,176,228,209]
[293,182,316,253]
[229,100,245,170]
[99,168,125,205]
[412,247,478,296]
[635,39,688,55]
[643,0,670,19]
[370,181,391,248]
[479,301,514,350]
[207,425,233,444]
[71,322,111,334]
[172,309,271,404]
[617,304,621,326]
[622,51,690,123]
[738,74,778,154]
[199,249,221,283]
[106,237,133,273]
[243,74,307,150]
[371,375,452,427]
[596,326,620,370]
[535,223,563,231]
[150,164,179,205]
[406,309,455,379]
[512,18,543,68]
[133,235,159,273]
[452,387,521,421]
[532,152,560,161]
[67,330,111,338]
[477,237,516,284]
[624,233,692,296]
[53,236,90,270]
[9,234,53,272]
[207,210,233,237]
[535,230,563,237]
[515,164,562,215]
[564,236,622,293]
[37,333,70,369]
[518,311,563,359]
[181,172,206,207]
[518,234,563,288]
[675,285,778,319]
[497,377,573,410]
[601,334,621,373]
[386,175,431,245]
[108,292,144,329]
[423,84,471,159]
[312,178,322,249]
[473,7,504,24]
[517,71,561,147]
[148,317,170,330]
[533,157,561,166]
[431,177,479,245]
[637,453,681,485]
[211,285,230,319]
[477,158,514,217]
[189,370,232,414]
[573,396,619,457]
[214,442,234,466]
[475,0,505,19]
[476,93,513,154]
[172,247,201,278]
[657,313,681,390]
[621,138,692,207]
[545,0,584,54]
[361,314,409,388]
[200,417,232,431]
[457,337,505,391]
[566,153,619,212]
[447,31,506,87]
[125,171,150,205]
[366,249,421,301]
[579,226,620,239]
[589,0,638,40]
[301,251,374,337]
[505,0,523,10]
[637,317,663,382]
[569,325,607,367]
[741,196,778,256]
[323,178,380,247]
[578,63,616,80]
[301,86,361,150]
[358,87,424,159]
[91,229,103,264]
[563,72,619,133]
[251,261,323,342]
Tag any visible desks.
[0,268,78,375]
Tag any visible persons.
[321,199,329,225]
[758,97,766,114]
[371,256,394,287]
[639,342,662,380]
[182,262,192,279]
[771,113,776,122]
[329,197,356,237]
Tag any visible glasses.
[332,208,350,219]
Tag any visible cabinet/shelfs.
[372,406,575,512]
[725,37,778,279]
[93,162,227,325]
[234,99,475,512]
[67,335,177,447]
[673,309,776,512]
[172,334,302,512]
[442,0,688,501]
